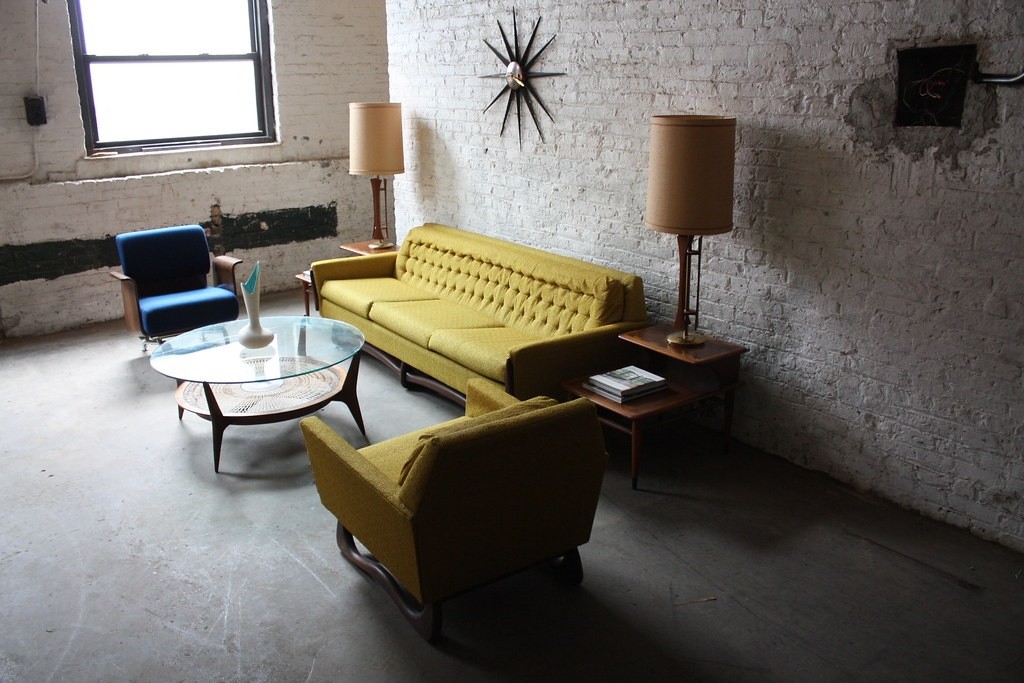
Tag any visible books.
[583,365,666,404]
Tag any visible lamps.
[348,101,405,250]
[645,114,735,346]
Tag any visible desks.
[294,238,401,316]
[555,322,747,489]
[150,316,366,473]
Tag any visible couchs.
[109,225,244,352]
[299,377,608,643]
[310,223,644,408]
